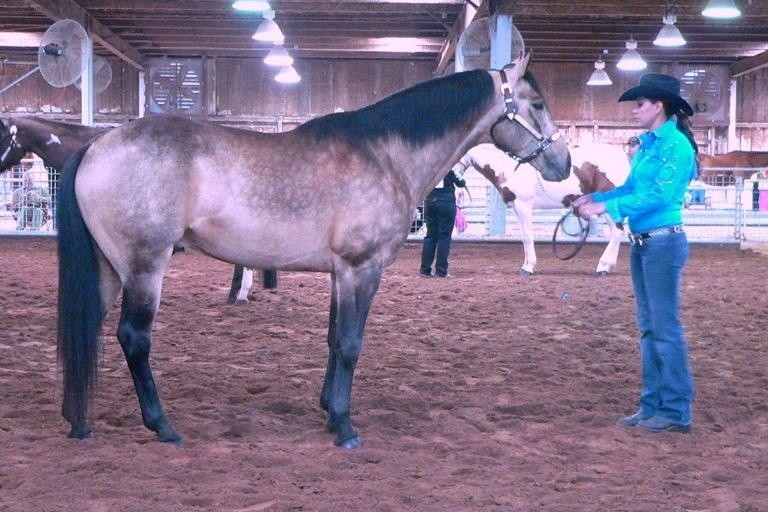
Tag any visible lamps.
[584,13,613,88]
[650,1,687,53]
[613,30,649,77]
[231,1,302,89]
[701,0,742,23]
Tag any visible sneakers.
[434,273,457,279]
[418,271,436,279]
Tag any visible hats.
[617,71,694,117]
[625,136,640,145]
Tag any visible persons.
[12,172,52,232]
[624,137,642,166]
[420,170,466,278]
[570,74,701,432]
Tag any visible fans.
[72,51,114,98]
[38,16,92,89]
[456,15,525,75]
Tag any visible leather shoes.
[638,415,693,434]
[616,407,656,428]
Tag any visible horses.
[451,142,633,276]
[1,114,278,306]
[696,150,768,187]
[54,47,572,452]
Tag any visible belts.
[626,225,685,242]
